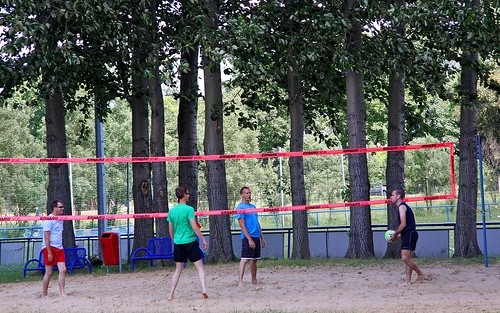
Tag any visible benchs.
[23,248,93,277]
[131,235,206,271]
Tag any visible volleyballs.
[384,230,400,243]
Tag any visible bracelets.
[395,231,399,235]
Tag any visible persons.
[167,186,209,301]
[388,188,426,287]
[236,187,266,288]
[43,199,68,300]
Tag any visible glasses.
[54,205,64,208]
[185,192,190,195]
